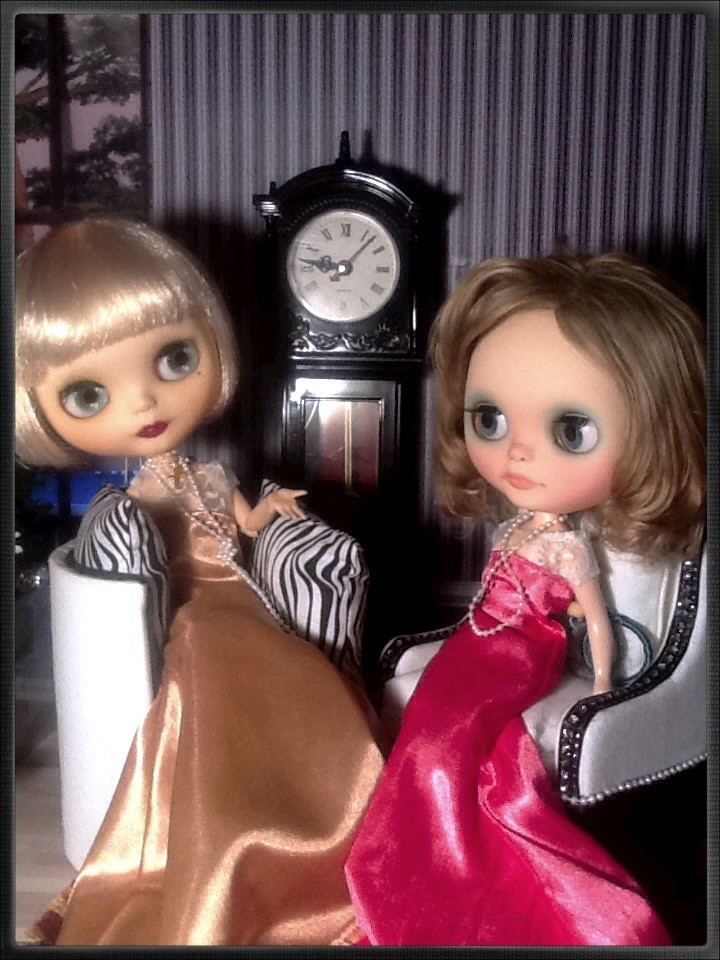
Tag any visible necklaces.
[469,511,566,639]
[143,451,297,639]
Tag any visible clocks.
[252,132,452,585]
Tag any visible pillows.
[248,479,369,682]
[73,485,178,661]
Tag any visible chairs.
[46,542,154,871]
[374,532,709,813]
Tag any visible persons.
[15,213,392,945]
[343,252,706,947]
